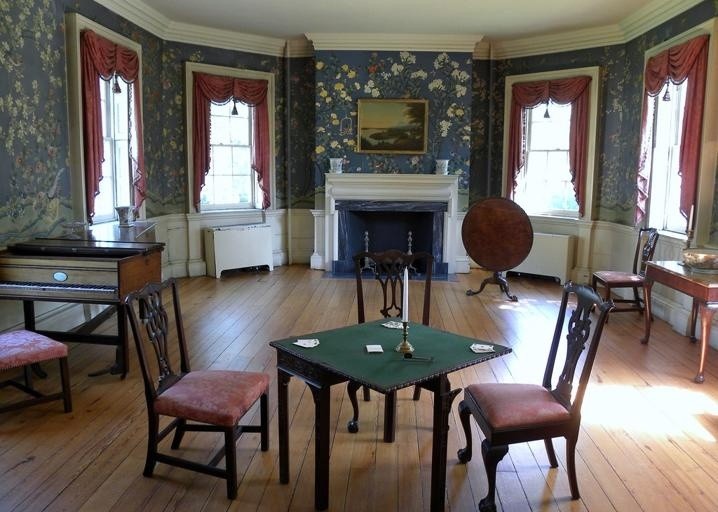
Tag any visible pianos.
[0,238,165,380]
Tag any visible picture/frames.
[357,98,428,156]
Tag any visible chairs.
[455,278,615,512]
[348,241,436,403]
[595,225,658,321]
[123,277,272,497]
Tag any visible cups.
[436,159,449,175]
[329,158,343,173]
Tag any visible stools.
[2,325,73,421]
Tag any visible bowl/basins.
[682,247,718,275]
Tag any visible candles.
[401,266,409,320]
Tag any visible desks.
[500,230,575,286]
[460,195,533,302]
[206,224,274,278]
[637,256,717,387]
[265,315,512,512]
